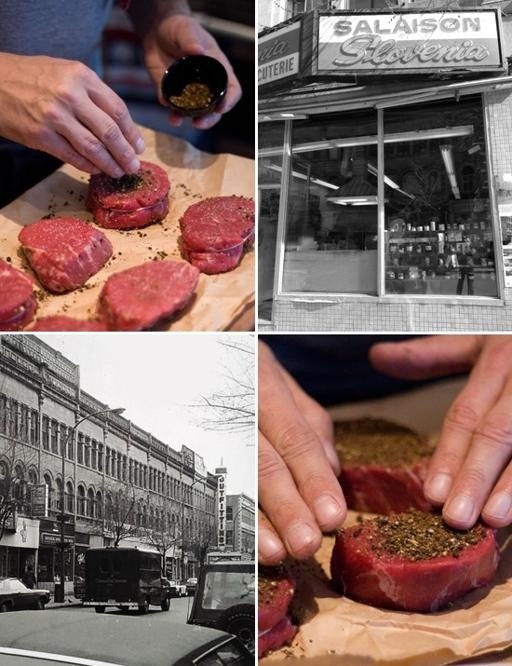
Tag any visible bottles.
[386,219,495,280]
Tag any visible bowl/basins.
[161,53,229,117]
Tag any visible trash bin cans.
[54,584,65,603]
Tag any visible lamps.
[439,144,460,198]
[265,163,339,191]
[384,176,399,190]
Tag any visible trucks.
[81,544,173,615]
[203,551,252,564]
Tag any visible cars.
[0,574,53,611]
[0,610,257,666]
[162,576,198,598]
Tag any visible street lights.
[179,474,223,583]
[52,405,127,605]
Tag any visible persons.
[0,0,243,207]
[259,335,512,563]
[457,237,477,295]
[22,564,37,590]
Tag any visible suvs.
[182,557,255,658]
